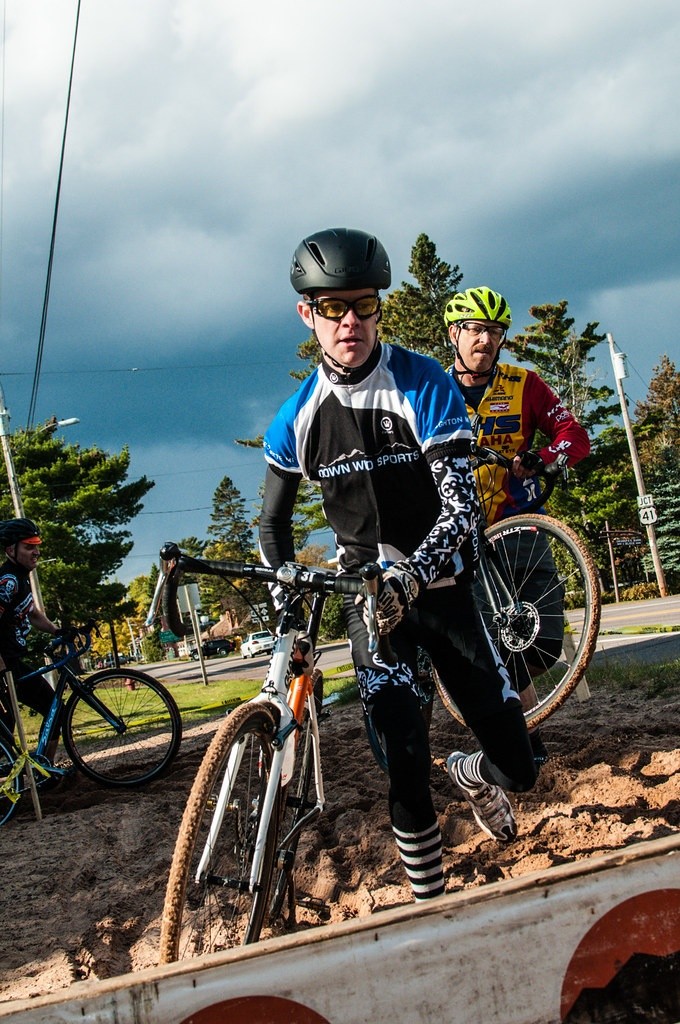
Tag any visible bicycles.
[144,540,436,966]
[0,626,181,829]
[436,412,605,734]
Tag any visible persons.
[444,287,591,765]
[258,228,538,905]
[0,518,80,792]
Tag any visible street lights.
[4,417,81,734]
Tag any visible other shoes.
[37,766,77,790]
[534,746,549,768]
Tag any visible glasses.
[306,295,381,321]
[452,320,506,343]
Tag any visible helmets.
[0,518,41,549]
[290,228,391,294]
[443,287,513,328]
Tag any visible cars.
[239,631,277,659]
[189,639,235,661]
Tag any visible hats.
[22,536,42,544]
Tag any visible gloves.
[353,567,419,636]
[56,624,78,644]
[0,669,17,695]
[274,606,314,678]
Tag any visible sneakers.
[447,751,517,844]
